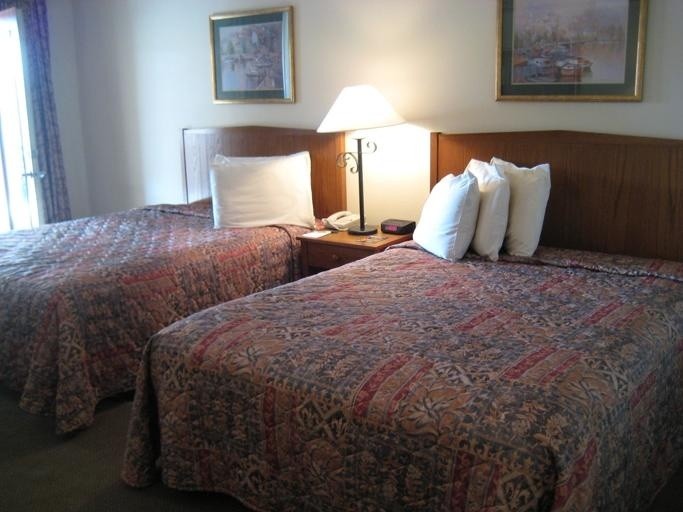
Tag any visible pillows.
[209,150,316,232]
[465,159,510,262]
[490,156,551,257]
[413,170,480,264]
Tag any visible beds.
[0,126,346,437]
[123,129,683,512]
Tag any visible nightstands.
[296,224,410,278]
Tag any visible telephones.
[324,211,366,230]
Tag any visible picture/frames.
[494,0,648,102]
[209,5,295,104]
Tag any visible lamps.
[317,84,405,235]
